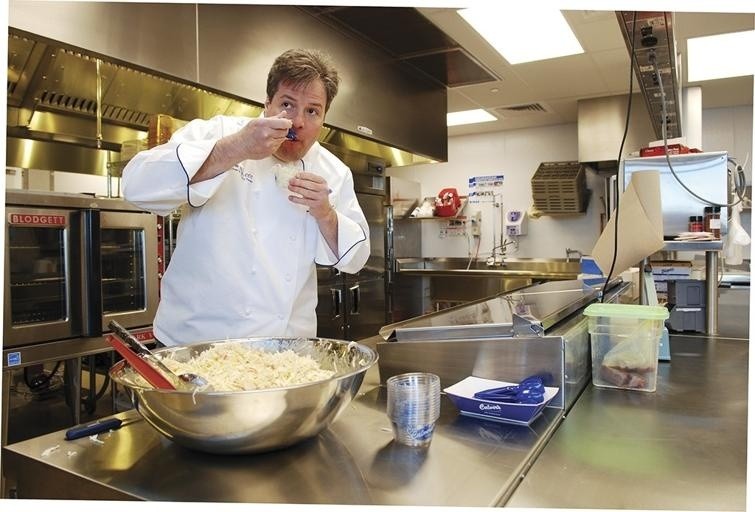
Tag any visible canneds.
[689,216,703,232]
[704,206,719,239]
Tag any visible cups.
[688,205,723,241]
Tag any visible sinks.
[395,257,583,279]
[501,276,623,294]
[379,293,605,336]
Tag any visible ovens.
[4,193,161,348]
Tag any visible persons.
[119,48,371,354]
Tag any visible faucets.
[563,247,585,265]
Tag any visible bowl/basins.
[105,336,382,458]
